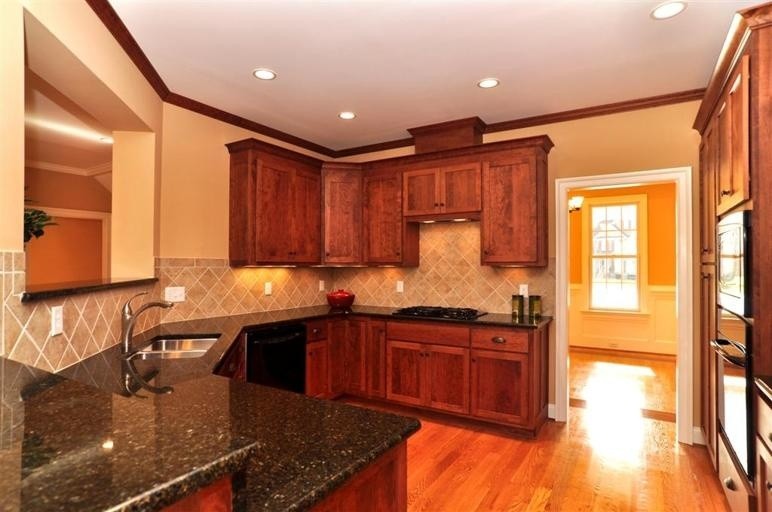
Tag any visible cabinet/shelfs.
[480,135,555,268]
[304,315,329,402]
[698,262,720,473]
[698,123,717,263]
[363,156,421,269]
[228,138,323,268]
[402,144,482,223]
[469,322,550,441]
[712,2,771,216]
[753,377,772,512]
[385,316,468,421]
[322,161,364,268]
[344,312,385,403]
[327,314,345,401]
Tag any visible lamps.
[568,195,585,213]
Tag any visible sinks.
[127,351,207,359]
[133,337,220,351]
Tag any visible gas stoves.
[395,306,477,322]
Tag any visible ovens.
[714,213,750,479]
[244,321,309,395]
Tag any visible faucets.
[120,291,174,354]
[119,359,174,401]
